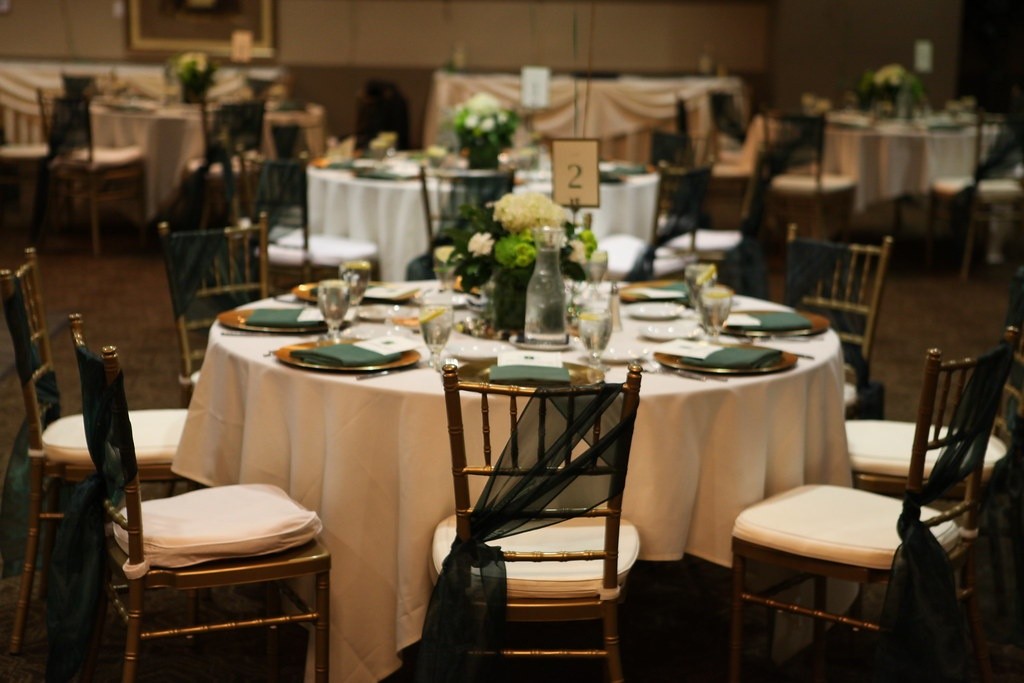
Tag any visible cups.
[432,249,456,284]
[370,132,396,160]
[684,264,717,306]
[581,249,608,287]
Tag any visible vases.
[492,273,525,338]
[468,148,500,169]
[178,85,207,102]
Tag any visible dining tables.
[172,282,847,683]
[306,150,665,279]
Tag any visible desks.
[424,69,740,160]
[89,96,320,237]
[754,112,994,248]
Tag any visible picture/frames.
[125,0,279,62]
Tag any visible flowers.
[451,93,522,149]
[174,52,216,89]
[872,64,930,108]
[444,191,598,297]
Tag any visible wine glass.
[418,294,454,368]
[698,286,732,340]
[340,260,370,326]
[578,295,613,373]
[317,277,350,341]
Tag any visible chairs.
[0,101,1024,683]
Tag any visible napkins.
[644,284,703,300]
[681,348,783,370]
[601,172,619,182]
[743,313,812,330]
[357,166,398,180]
[362,287,422,300]
[246,308,319,327]
[613,166,643,174]
[491,364,572,386]
[291,346,402,368]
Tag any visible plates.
[583,350,652,363]
[293,281,383,303]
[721,311,829,337]
[457,358,604,387]
[619,279,689,301]
[654,342,797,373]
[411,291,467,307]
[219,308,352,332]
[625,303,686,320]
[357,306,427,322]
[275,340,420,370]
[637,322,700,341]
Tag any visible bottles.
[523,227,570,352]
[579,212,598,256]
